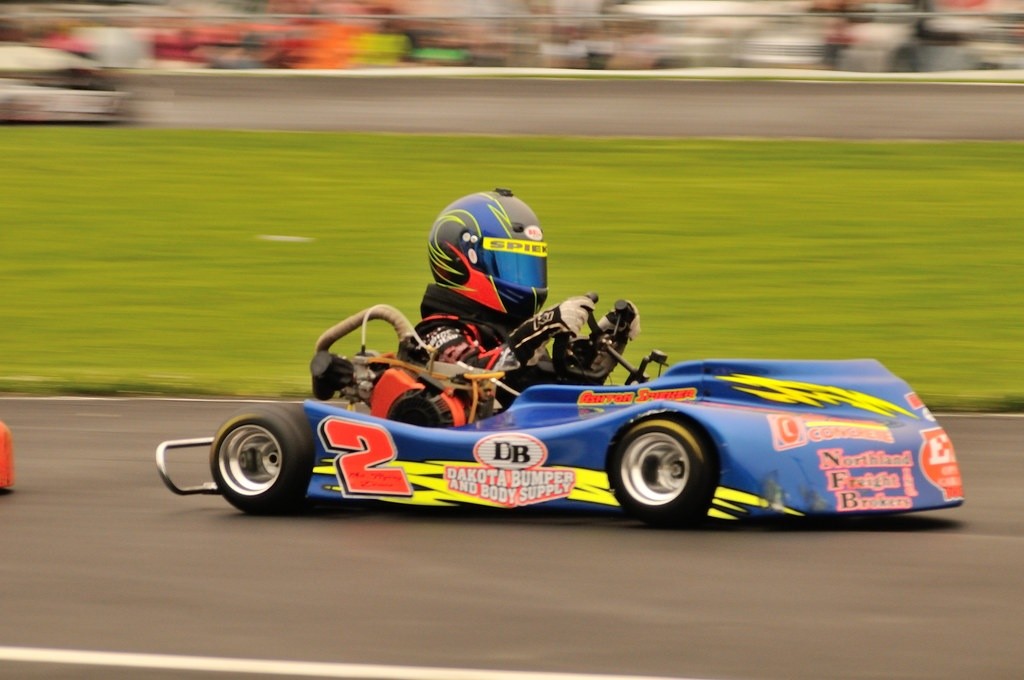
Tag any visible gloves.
[508,296,595,365]
[589,301,640,370]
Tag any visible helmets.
[429,189,548,326]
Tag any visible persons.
[397,187,641,424]
